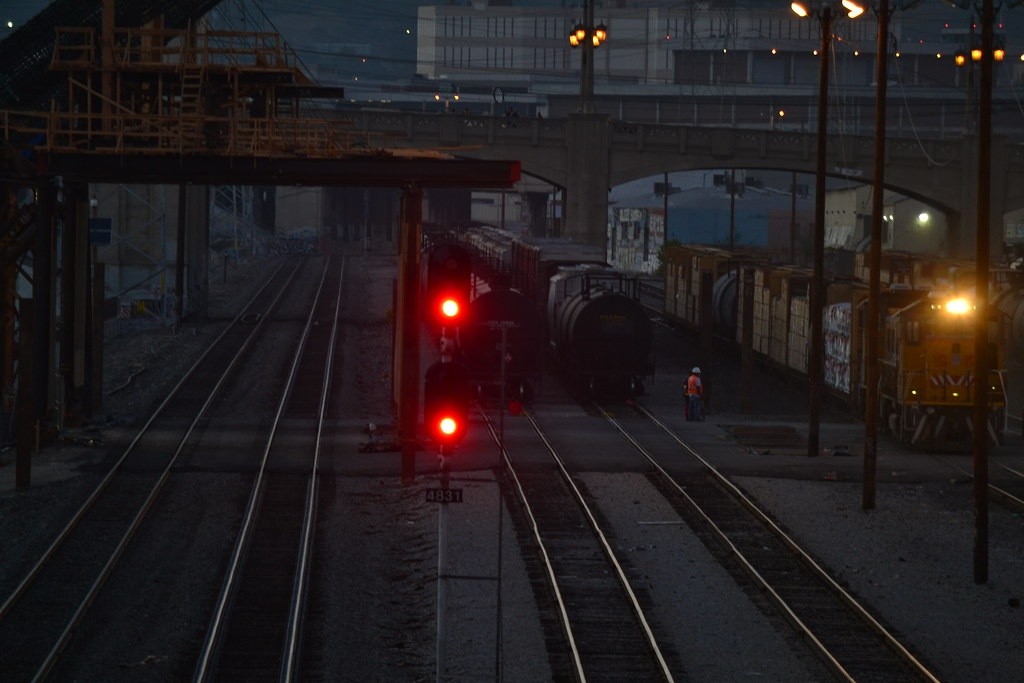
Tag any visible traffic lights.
[426,238,472,340]
[424,361,471,449]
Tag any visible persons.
[687,367,705,420]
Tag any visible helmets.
[692,367,701,373]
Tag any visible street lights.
[955,0,1007,580]
[567,18,608,114]
[793,1,908,509]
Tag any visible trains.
[662,243,1008,453]
[462,225,655,399]
